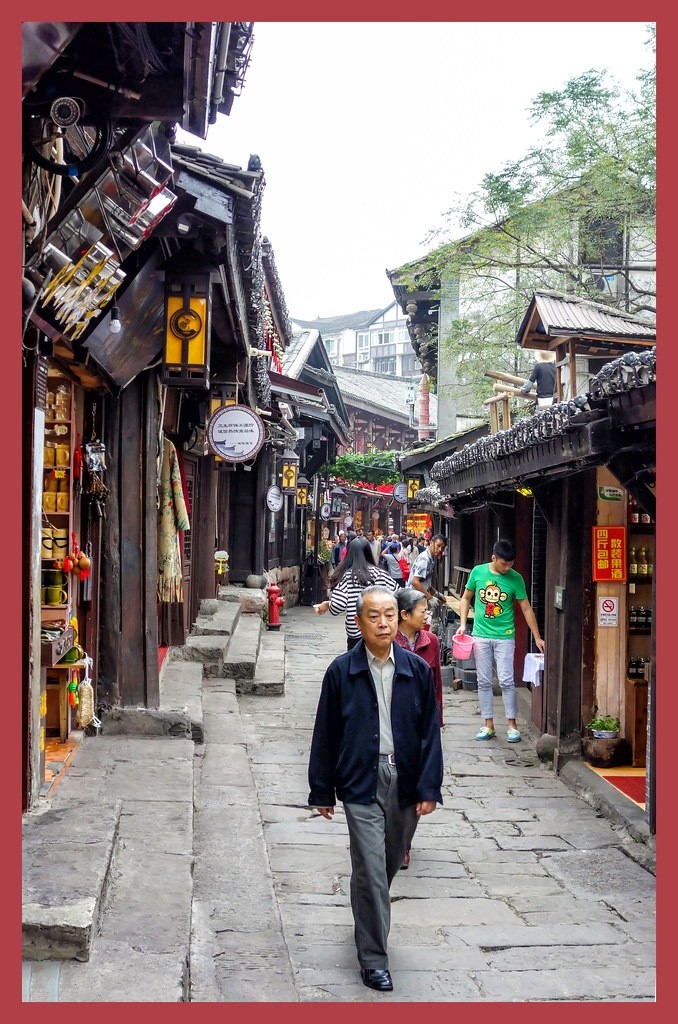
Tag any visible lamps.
[330,487,344,515]
[297,473,310,509]
[428,310,439,315]
[161,244,213,390]
[281,448,300,495]
[407,475,420,500]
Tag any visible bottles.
[630,547,653,574]
[628,657,650,678]
[630,498,654,523]
[629,606,652,627]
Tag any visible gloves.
[434,591,447,604]
[429,596,441,608]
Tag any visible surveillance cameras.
[278,401,294,419]
[41,92,86,128]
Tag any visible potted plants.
[585,714,621,739]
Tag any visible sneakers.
[400,850,410,869]
[507,726,521,742]
[475,727,497,741]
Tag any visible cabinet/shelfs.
[626,678,649,768]
[40,372,76,736]
[628,523,656,636]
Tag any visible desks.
[522,653,544,731]
[42,659,86,744]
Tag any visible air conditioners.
[582,273,618,298]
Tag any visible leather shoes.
[360,967,393,991]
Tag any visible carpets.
[602,776,646,803]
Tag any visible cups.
[46,393,69,419]
[42,529,67,559]
[44,443,69,467]
[43,474,68,513]
[40,569,68,605]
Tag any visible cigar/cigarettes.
[309,813,322,818]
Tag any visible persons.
[312,537,402,651]
[331,527,433,588]
[520,350,556,410]
[405,534,448,609]
[456,540,545,743]
[308,586,444,992]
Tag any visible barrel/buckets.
[452,634,475,660]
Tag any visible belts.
[379,754,396,766]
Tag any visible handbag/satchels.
[399,559,410,581]
